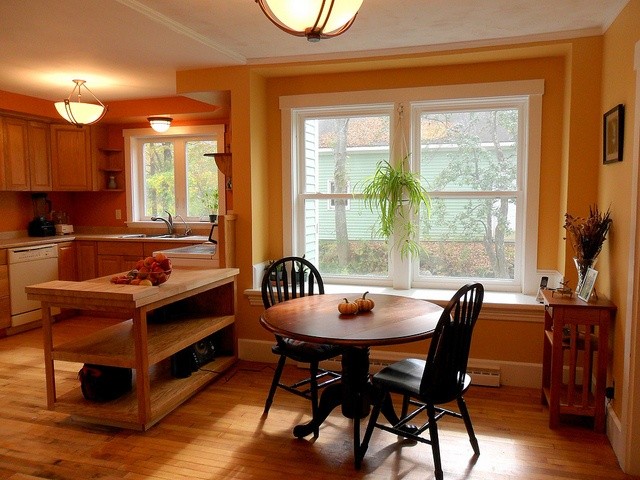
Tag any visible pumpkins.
[354,292,375,313]
[338,297,357,316]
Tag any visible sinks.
[159,234,190,238]
[119,233,167,238]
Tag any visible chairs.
[360,278,484,480]
[261,256,346,439]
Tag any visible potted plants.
[292,254,308,287]
[263,259,285,287]
[351,153,433,263]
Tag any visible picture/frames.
[603,104,624,164]
[578,267,598,303]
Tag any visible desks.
[259,291,454,438]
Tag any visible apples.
[135,260,144,269]
[138,266,149,279]
[161,259,172,270]
[151,262,164,276]
[153,252,168,262]
[144,256,154,269]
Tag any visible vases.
[572,256,598,294]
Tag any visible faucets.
[174,214,192,235]
[151,211,173,233]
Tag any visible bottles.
[109,176,116,188]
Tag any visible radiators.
[295,354,502,387]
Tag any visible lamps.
[254,0,365,42]
[146,116,173,133]
[54,78,109,129]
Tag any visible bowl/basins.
[129,271,171,285]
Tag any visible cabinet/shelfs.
[143,243,195,264]
[58,240,76,314]
[97,242,142,278]
[537,288,617,432]
[49,122,96,192]
[25,267,241,432]
[96,124,125,192]
[75,242,97,283]
[0,114,53,193]
[203,152,232,191]
[1,248,12,330]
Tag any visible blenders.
[29,193,56,236]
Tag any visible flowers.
[563,201,615,287]
